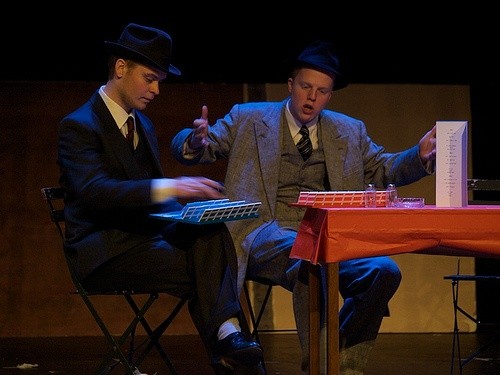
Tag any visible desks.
[289,204,500,375]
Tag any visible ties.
[126,116,135,150]
[296,125,313,162]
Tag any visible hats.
[104,23,181,75]
[294,44,353,91]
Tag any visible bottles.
[364,184,376,208]
[386,184,396,208]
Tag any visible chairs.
[242,272,280,375]
[40,187,189,375]
[443,256,500,375]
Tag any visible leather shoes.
[208,332,264,375]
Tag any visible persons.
[171,49,436,375]
[58,22,266,375]
